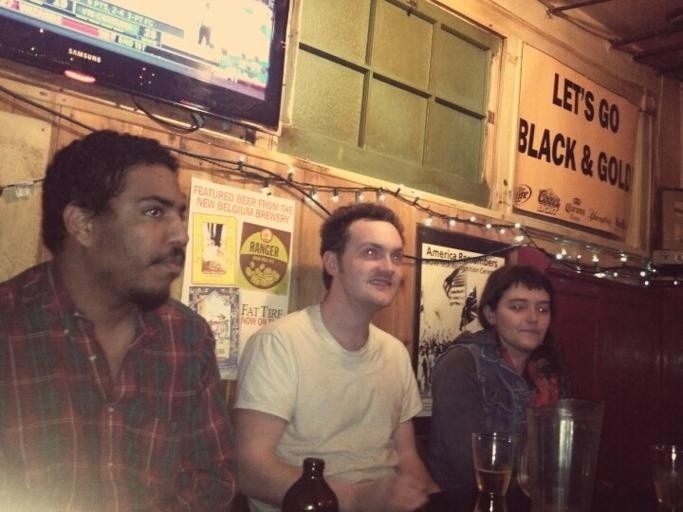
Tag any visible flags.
[459,286,478,331]
[442,265,465,305]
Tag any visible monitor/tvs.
[0,0,292,136]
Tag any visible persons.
[417,342,445,397]
[230,202,442,512]
[0,132,238,512]
[423,263,571,491]
[220,48,259,83]
[198,3,211,46]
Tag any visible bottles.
[279,457,338,512]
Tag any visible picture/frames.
[411,224,513,434]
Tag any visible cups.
[472,397,603,511]
[651,442,683,512]
